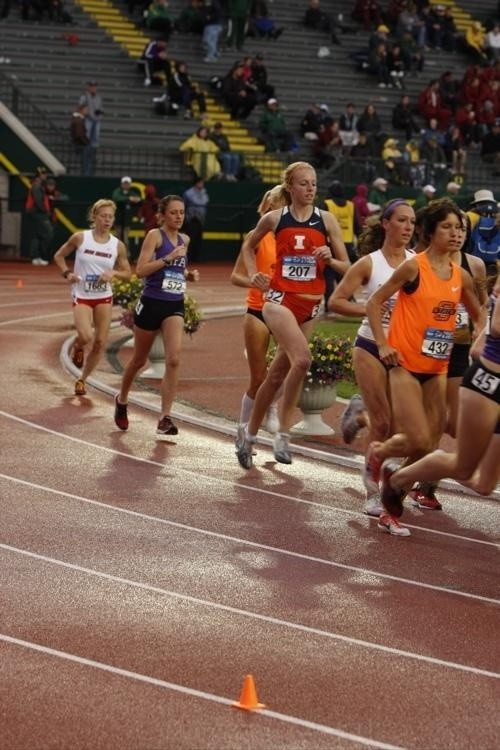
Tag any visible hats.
[35,166,56,184]
[377,25,390,33]
[267,98,278,105]
[467,189,497,204]
[120,175,132,184]
[371,135,463,195]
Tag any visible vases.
[289,380,336,436]
[138,331,166,379]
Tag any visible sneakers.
[155,414,179,435]
[71,336,85,369]
[361,438,411,536]
[74,376,89,395]
[272,432,292,465]
[114,392,130,430]
[235,423,255,469]
[409,483,444,509]
[338,392,370,445]
[32,258,49,266]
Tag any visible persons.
[228,160,497,539]
[22,0,499,319]
[52,200,132,395]
[114,195,199,436]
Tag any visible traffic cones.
[228,673,266,712]
[15,279,24,289]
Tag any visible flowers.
[120,291,200,338]
[267,332,357,393]
